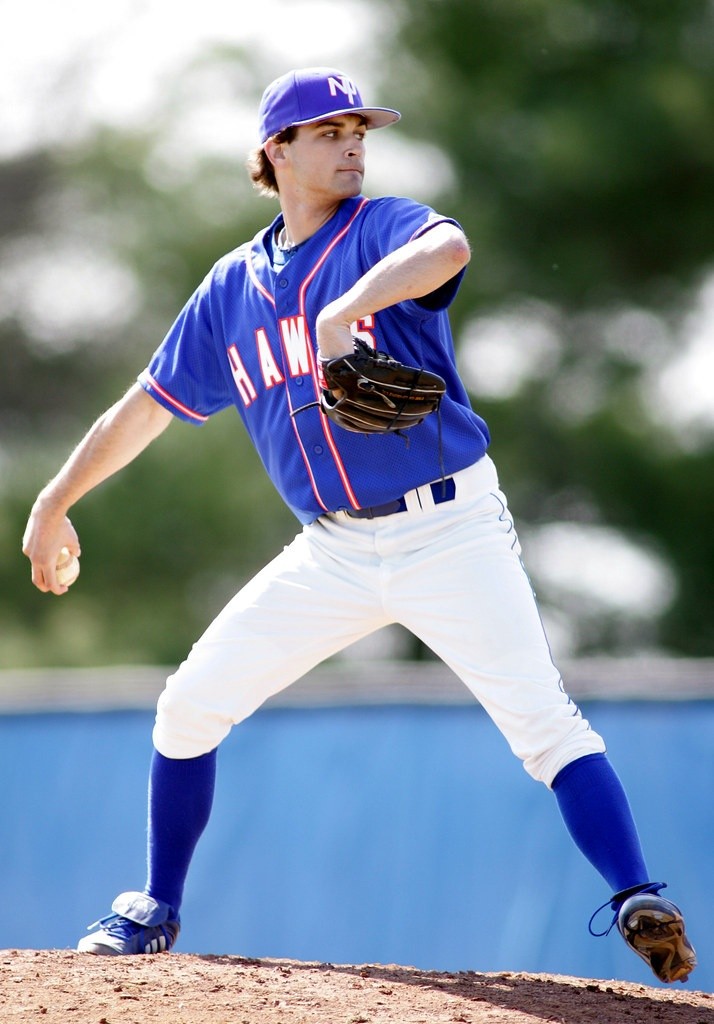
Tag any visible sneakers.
[589,882,698,983]
[77,891,181,956]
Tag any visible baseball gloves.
[318,337,449,438]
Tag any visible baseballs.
[48,548,82,592]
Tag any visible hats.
[257,67,402,144]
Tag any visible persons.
[23,69,698,983]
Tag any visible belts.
[346,477,456,519]
